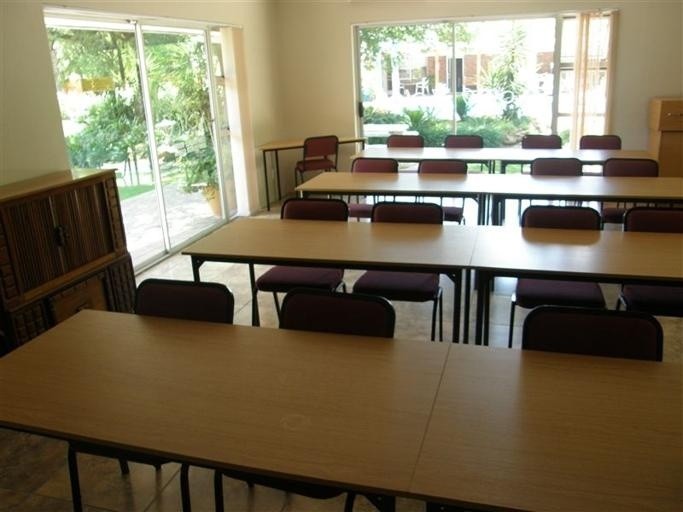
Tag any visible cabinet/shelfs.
[0,167,137,358]
[648,98,683,178]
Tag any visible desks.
[409,341,682,512]
[0,308,452,512]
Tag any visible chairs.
[214,287,394,512]
[66,279,234,511]
